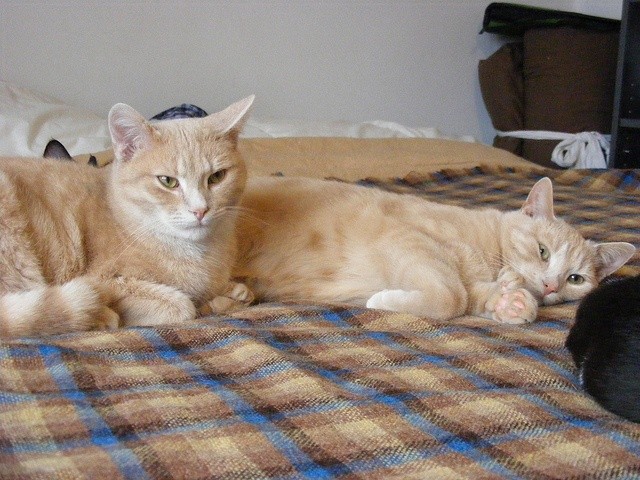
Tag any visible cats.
[565,273,640,423]
[198,175,636,327]
[0,94,266,339]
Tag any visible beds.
[0,78,639,480]
